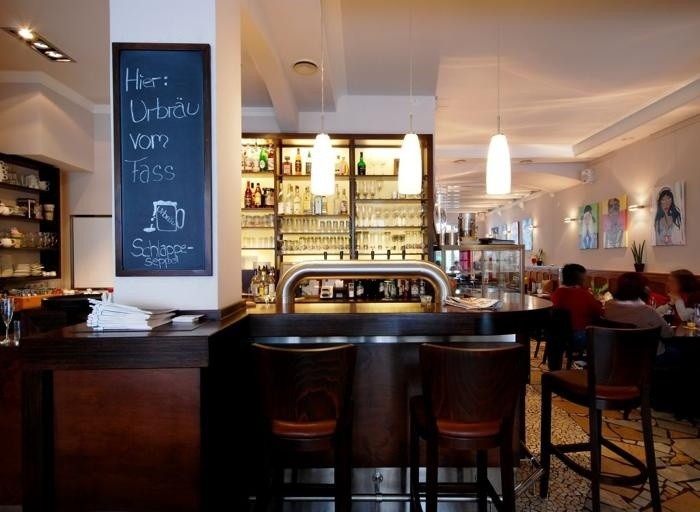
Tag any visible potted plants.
[535,248,545,266]
[630,239,646,272]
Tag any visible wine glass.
[0,298,16,345]
[355,205,427,227]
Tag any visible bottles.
[251,262,275,303]
[305,151,313,174]
[334,183,341,214]
[378,278,425,298]
[341,156,349,176]
[283,156,292,175]
[285,183,293,214]
[334,155,341,176]
[259,145,267,172]
[303,187,313,215]
[357,152,366,176]
[245,181,253,207]
[254,183,262,207]
[294,186,301,214]
[268,142,276,172]
[277,182,285,214]
[356,281,364,299]
[250,182,255,208]
[295,148,302,175]
[341,189,348,214]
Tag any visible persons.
[603,198,625,248]
[604,272,675,343]
[549,263,607,354]
[655,269,700,328]
[578,205,597,250]
[653,190,683,244]
[449,261,463,272]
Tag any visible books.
[85,303,177,331]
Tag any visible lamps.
[628,204,645,211]
[310,1,335,196]
[398,1,422,195]
[293,61,318,77]
[486,0,511,195]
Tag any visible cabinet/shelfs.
[0,153,61,280]
[241,132,433,303]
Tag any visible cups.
[44,212,55,220]
[355,179,422,199]
[355,231,423,249]
[22,232,57,249]
[279,218,351,233]
[240,213,273,226]
[242,236,274,248]
[39,185,50,192]
[153,200,186,232]
[2,206,13,216]
[280,236,351,251]
[43,204,55,212]
[39,181,49,186]
[1,238,16,249]
[0,160,8,182]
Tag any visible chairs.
[534,308,587,370]
[598,319,668,420]
[410,345,520,511]
[538,327,661,512]
[250,342,356,511]
